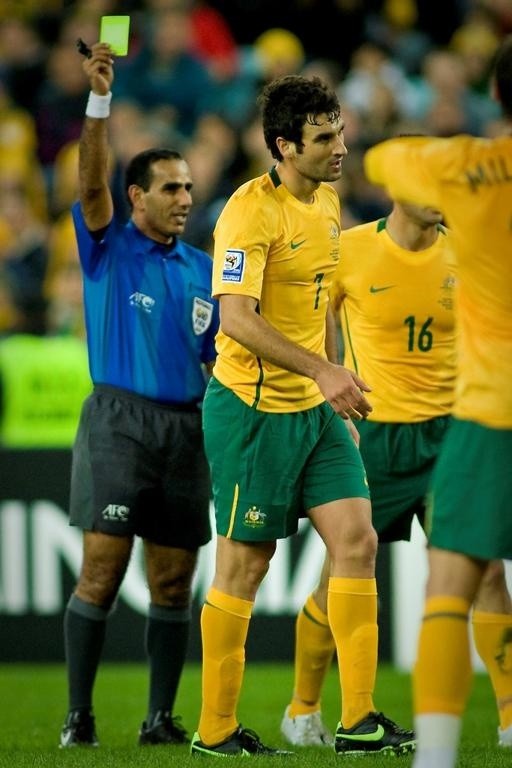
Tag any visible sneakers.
[61,716,100,747]
[332,711,416,757]
[188,721,296,759]
[138,717,190,745]
[280,703,333,747]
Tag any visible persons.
[277,133,510,745]
[0,2,512,338]
[359,39,509,768]
[53,38,220,754]
[187,76,425,759]
